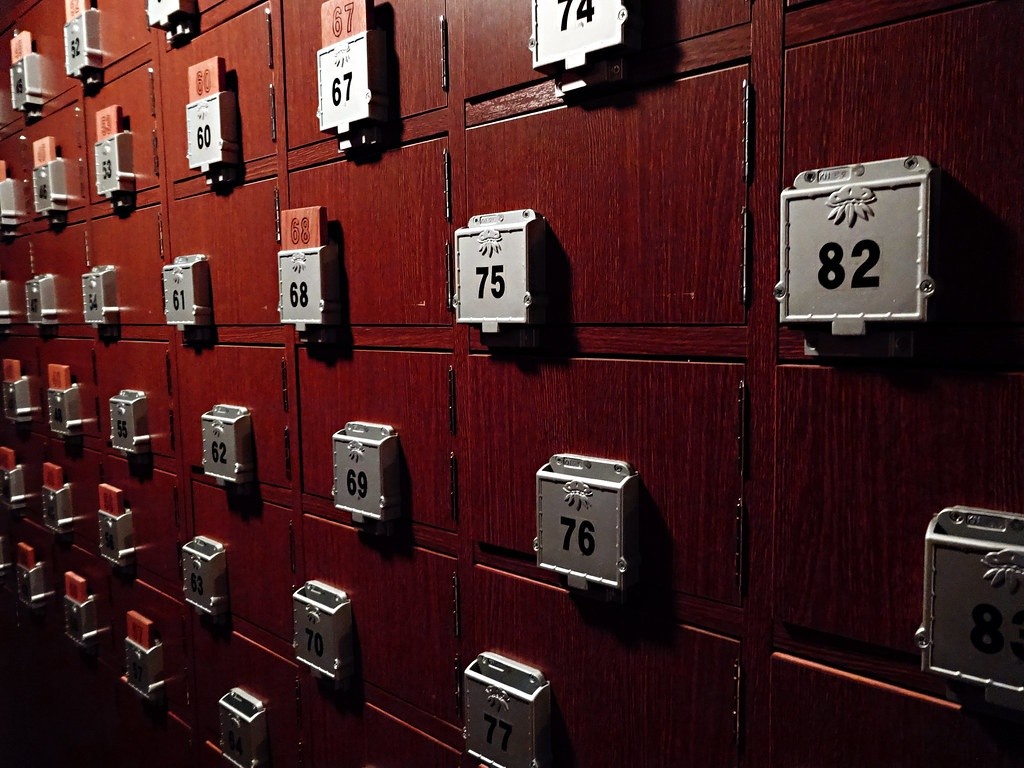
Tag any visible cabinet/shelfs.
[0,0,1024,768]
